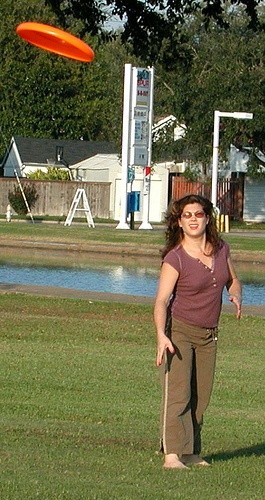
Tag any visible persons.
[153,194,242,469]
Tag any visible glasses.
[181,211,205,219]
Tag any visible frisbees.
[17,22,94,63]
